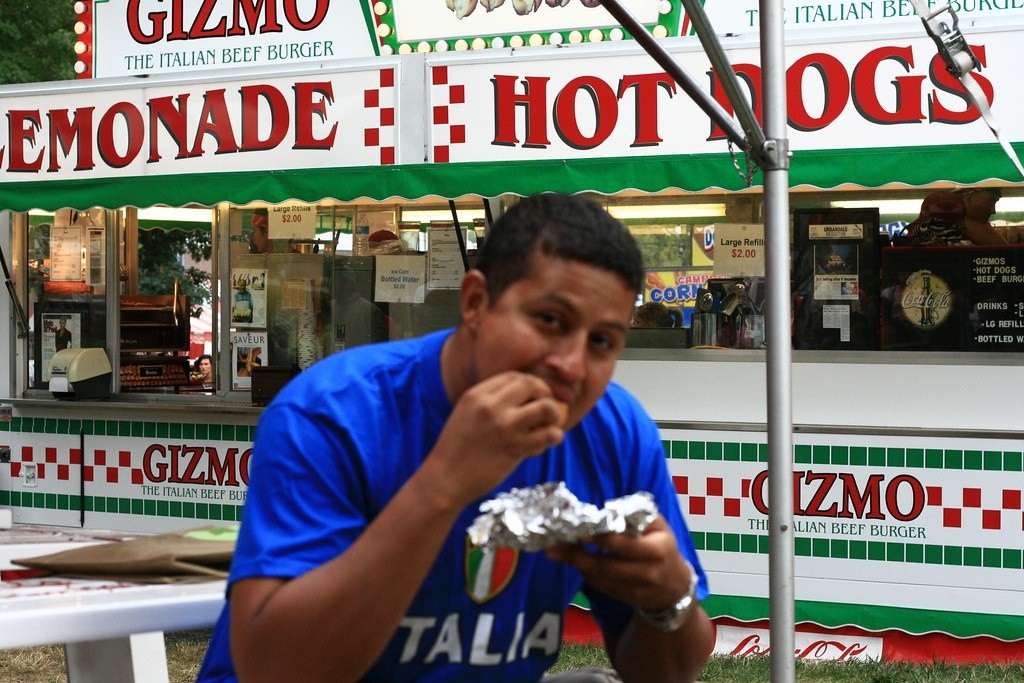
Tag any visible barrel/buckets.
[730,313,765,349]
[691,312,733,349]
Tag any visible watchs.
[632,557,701,631]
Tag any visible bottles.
[354,226,370,255]
[334,324,346,353]
[921,274,934,326]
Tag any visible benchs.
[0,579,230,683]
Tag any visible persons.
[631,300,684,329]
[233,278,253,324]
[914,185,1011,246]
[188,353,214,384]
[248,208,275,253]
[235,344,261,376]
[316,251,401,355]
[54,318,72,354]
[187,189,718,682]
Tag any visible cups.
[297,276,325,369]
[296,243,314,255]
[429,219,454,229]
[473,218,486,248]
[397,220,420,252]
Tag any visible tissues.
[46,347,113,401]
